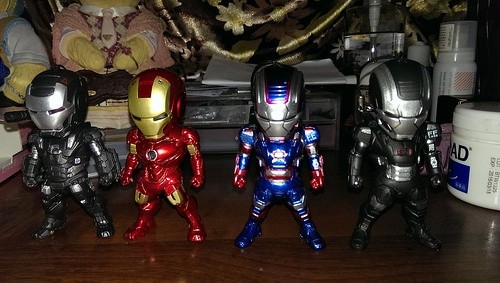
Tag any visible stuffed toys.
[1,0,175,104]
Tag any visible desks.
[0,153,500,283]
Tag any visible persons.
[346,59,444,252]
[232,62,327,251]
[21,69,122,239]
[121,68,207,241]
